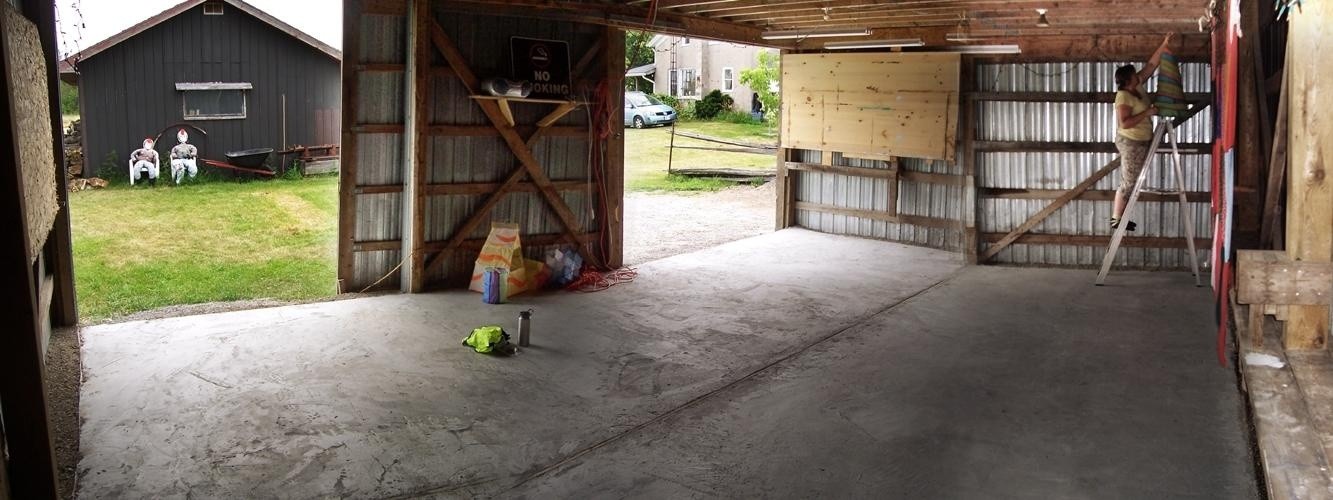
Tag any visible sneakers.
[1109,215,1137,231]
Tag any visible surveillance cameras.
[64,51,69,59]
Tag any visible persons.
[171,128,198,184]
[130,139,156,186]
[1110,29,1176,231]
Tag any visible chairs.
[128,149,161,186]
[169,144,197,182]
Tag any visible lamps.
[939,44,1025,58]
[821,38,925,54]
[1036,10,1049,30]
[758,18,874,40]
[820,7,835,21]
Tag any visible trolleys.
[198,146,277,182]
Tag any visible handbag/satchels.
[460,323,513,355]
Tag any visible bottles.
[516,309,535,348]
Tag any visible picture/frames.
[508,36,572,100]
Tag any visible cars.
[623,89,676,131]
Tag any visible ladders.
[1094,115,1202,288]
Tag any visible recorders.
[481,75,536,98]
